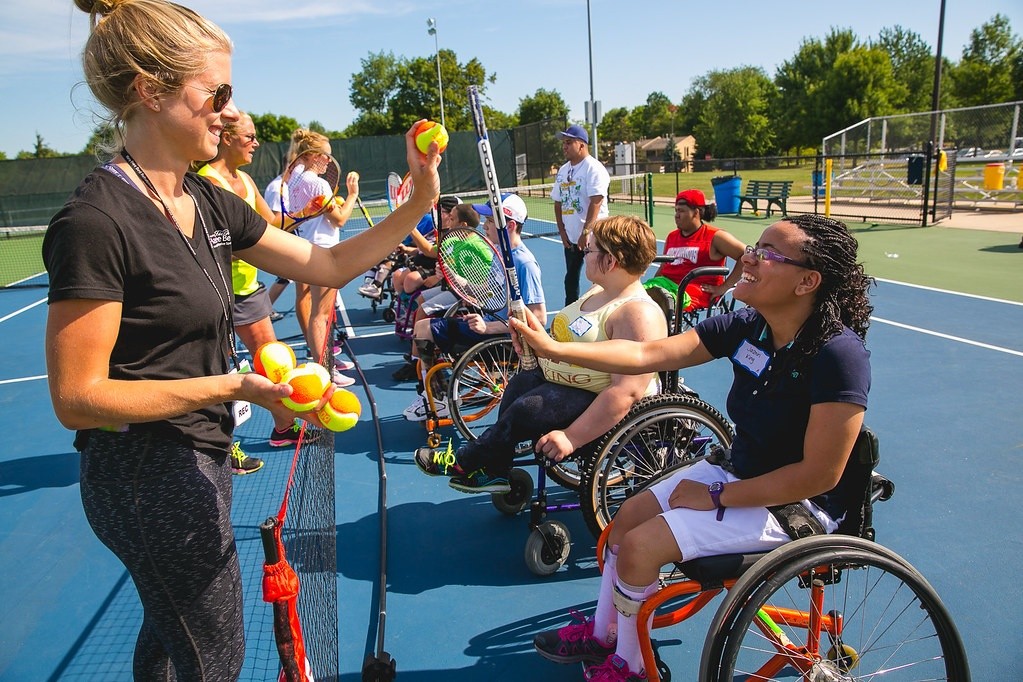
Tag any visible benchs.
[735,179,794,218]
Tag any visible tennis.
[254,341,296,383]
[415,122,450,155]
[318,389,362,432]
[334,196,345,208]
[280,370,324,411]
[295,362,331,391]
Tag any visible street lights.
[428,17,452,193]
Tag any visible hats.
[675,189,705,206]
[558,125,588,144]
[471,193,527,225]
[439,195,463,211]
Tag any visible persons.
[534,214,877,682]
[642,189,747,313]
[196,111,335,476]
[264,128,359,388]
[414,214,669,495]
[356,193,547,420]
[550,125,610,306]
[41,3,443,682]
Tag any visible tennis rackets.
[396,171,416,206]
[437,224,513,326]
[384,171,402,213]
[431,195,443,228]
[355,196,375,227]
[467,85,538,371]
[280,147,341,231]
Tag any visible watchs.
[582,228,591,235]
[708,481,726,522]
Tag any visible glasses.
[744,245,819,271]
[584,247,612,255]
[161,80,232,113]
[317,149,332,159]
[567,167,573,182]
[231,132,257,142]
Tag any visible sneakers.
[449,466,511,494]
[391,354,418,382]
[358,279,382,298]
[332,365,355,387]
[533,609,618,664]
[306,346,342,360]
[331,357,355,371]
[402,390,463,422]
[333,340,344,347]
[414,438,465,476]
[269,418,322,446]
[228,441,264,475]
[583,654,649,682]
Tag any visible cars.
[956,147,984,157]
[984,149,1004,158]
[1004,147,1023,158]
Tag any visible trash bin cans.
[907,154,925,184]
[983,163,1005,191]
[710,175,744,213]
[812,170,833,196]
[1017,167,1023,191]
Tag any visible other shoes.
[269,311,284,320]
[399,307,414,325]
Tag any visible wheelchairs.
[647,267,740,335]
[486,253,741,580]
[359,242,558,460]
[579,419,971,682]
